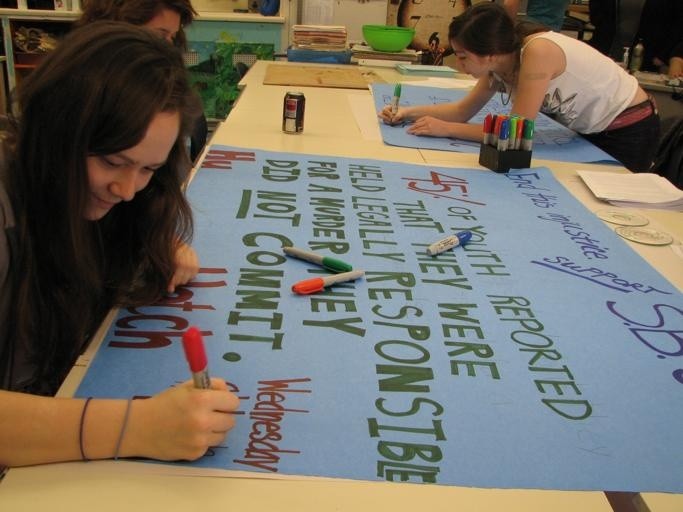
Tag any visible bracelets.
[79,396,94,462]
[112,398,132,459]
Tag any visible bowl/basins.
[361,24,415,53]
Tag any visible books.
[351,44,423,68]
[290,25,348,51]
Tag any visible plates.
[615,228,673,245]
[596,208,648,226]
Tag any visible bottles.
[630,38,645,71]
[622,47,630,69]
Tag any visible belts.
[607,91,658,131]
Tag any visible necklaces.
[500,66,516,105]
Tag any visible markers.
[183,327,214,389]
[426,230,472,256]
[292,270,365,295]
[392,83,401,113]
[483,112,535,151]
[284,247,352,273]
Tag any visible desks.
[354,57,682,512]
[0,55,617,510]
[628,66,683,150]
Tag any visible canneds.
[282,90,306,134]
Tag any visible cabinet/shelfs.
[0,0,299,151]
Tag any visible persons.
[377,0,661,173]
[78,0,198,47]
[422,37,454,66]
[0,21,241,469]
